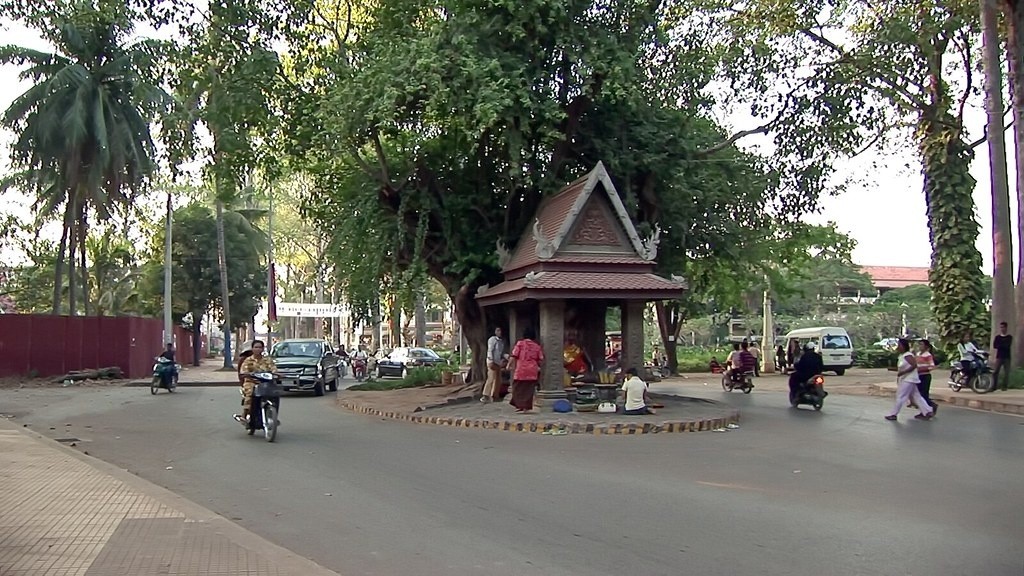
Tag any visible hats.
[239,340,253,356]
[806,341,815,349]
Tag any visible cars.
[366,346,450,380]
[272,337,342,396]
[872,338,899,347]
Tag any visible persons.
[723,338,760,389]
[480,327,504,403]
[958,333,989,387]
[621,368,656,415]
[652,345,664,366]
[506,330,543,412]
[605,343,622,378]
[988,321,1013,392]
[916,339,939,413]
[238,339,282,426]
[775,345,788,374]
[336,345,350,377]
[157,343,179,386]
[348,345,368,378]
[884,338,935,422]
[791,341,823,404]
[710,356,721,370]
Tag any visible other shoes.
[926,412,934,420]
[242,409,250,419]
[648,407,656,414]
[907,404,918,409]
[885,415,897,421]
[932,403,937,414]
[915,413,926,419]
[479,396,489,403]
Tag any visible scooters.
[150,356,182,395]
[351,358,367,382]
[946,348,996,394]
[720,362,755,394]
[232,371,281,442]
[793,374,828,411]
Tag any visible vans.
[775,327,856,376]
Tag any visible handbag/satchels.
[553,400,573,412]
[597,403,616,413]
